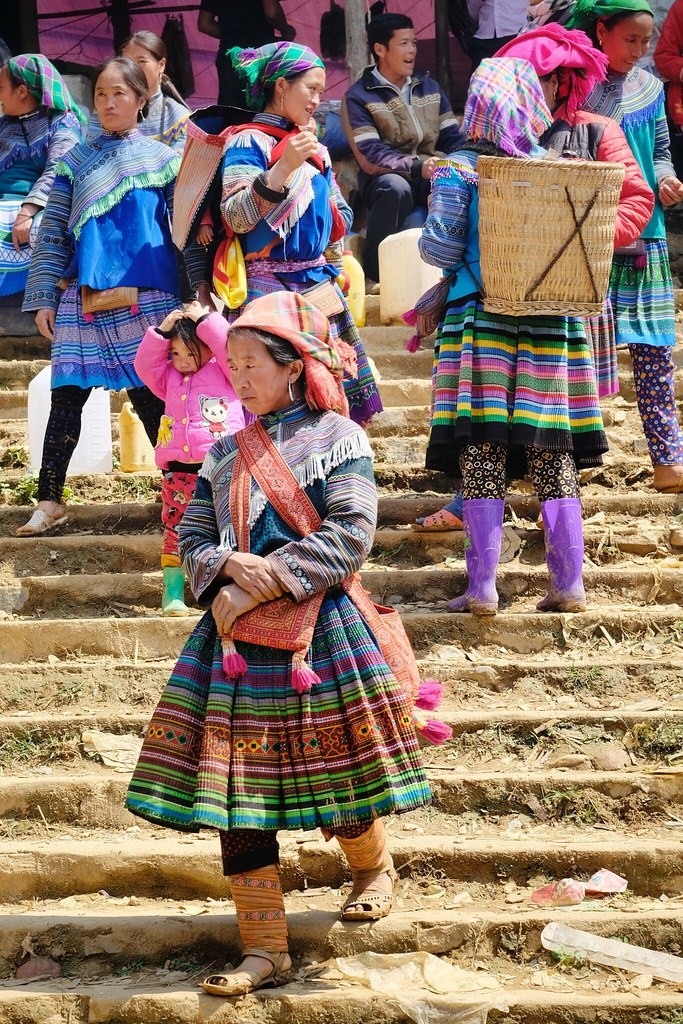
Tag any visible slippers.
[661,465,683,494]
[412,505,469,531]
[13,505,68,537]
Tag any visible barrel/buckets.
[378,227,445,326]
[28,363,113,478]
[120,401,157,472]
[336,250,366,328]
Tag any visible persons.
[121,289,432,996]
[197,0,296,107]
[216,42,383,425]
[0,52,85,343]
[16,31,192,534]
[340,13,463,294]
[465,1,683,493]
[402,20,654,614]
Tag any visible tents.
[0,0,683,103]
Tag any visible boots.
[161,567,188,617]
[442,496,501,618]
[536,496,587,614]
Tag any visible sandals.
[202,952,293,997]
[342,870,399,920]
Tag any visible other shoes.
[365,277,381,295]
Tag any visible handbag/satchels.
[212,232,245,310]
[81,287,139,321]
[364,602,424,704]
[299,277,343,319]
[219,591,326,692]
[402,271,457,353]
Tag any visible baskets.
[475,153,626,317]
